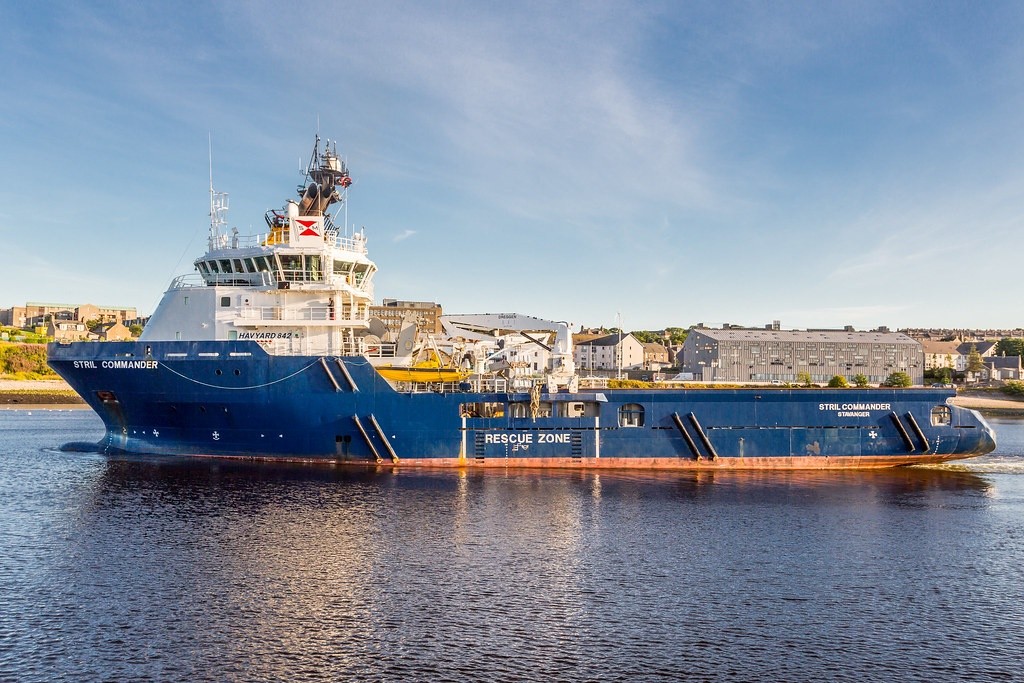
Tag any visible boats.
[374,331,475,383]
[47,133,997,472]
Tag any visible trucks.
[614,372,628,380]
[672,373,693,381]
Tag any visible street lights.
[618,312,621,380]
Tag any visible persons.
[327,298,334,320]
[938,415,942,423]
[459,354,473,392]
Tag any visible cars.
[770,380,787,385]
[641,375,649,382]
[932,383,957,388]
